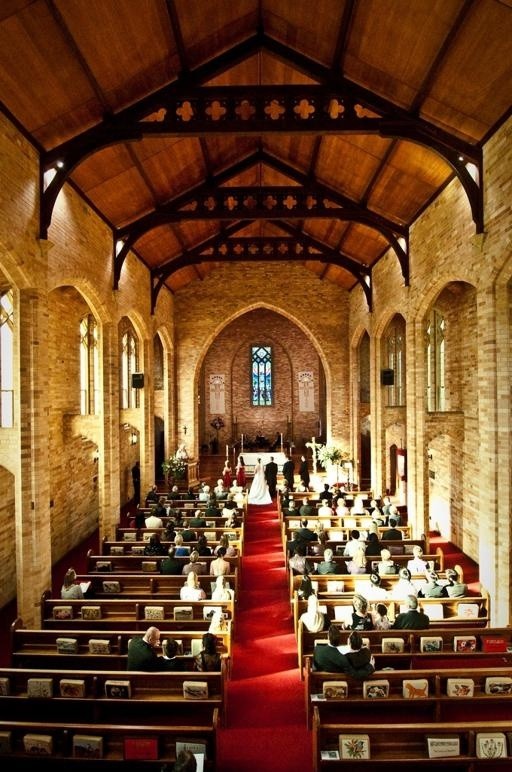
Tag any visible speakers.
[132,374,144,388]
[382,369,394,385]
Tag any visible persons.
[60,569,82,600]
[256,430,268,449]
[157,639,183,671]
[193,633,222,672]
[173,750,197,772]
[208,612,228,633]
[127,626,161,671]
[222,455,310,505]
[176,444,188,459]
[131,479,245,601]
[269,431,281,451]
[279,476,469,679]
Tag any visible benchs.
[275,489,512,771]
[0,490,249,771]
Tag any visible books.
[0,731,14,754]
[72,735,104,758]
[122,737,159,761]
[55,638,78,655]
[203,606,222,620]
[484,676,512,696]
[144,607,165,620]
[456,603,479,619]
[53,606,73,620]
[161,640,183,655]
[0,677,11,696]
[110,546,125,555]
[102,580,120,594]
[319,749,338,762]
[448,680,477,698]
[174,606,193,620]
[190,639,204,655]
[322,681,348,700]
[78,581,91,594]
[363,680,390,699]
[477,733,508,760]
[183,682,209,700]
[96,561,113,572]
[123,533,137,542]
[60,680,85,698]
[403,680,428,698]
[89,639,110,654]
[310,692,325,703]
[26,678,53,698]
[338,734,371,761]
[81,606,101,620]
[453,635,478,654]
[422,735,461,758]
[23,734,52,755]
[104,680,131,699]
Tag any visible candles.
[234,415,237,424]
[288,415,290,423]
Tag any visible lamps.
[131,433,138,445]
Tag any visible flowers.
[161,455,188,479]
